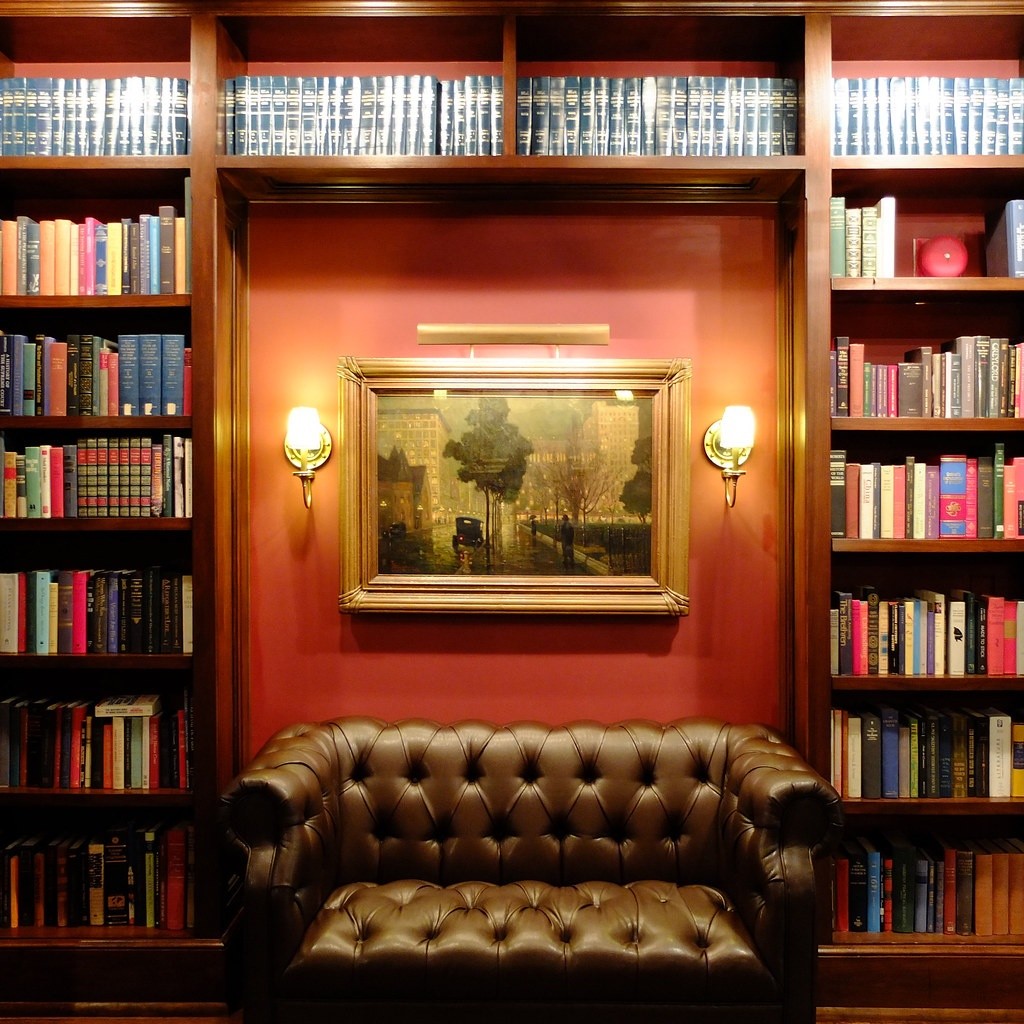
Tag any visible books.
[0,825,200,932]
[1,694,196,789]
[517,77,799,156]
[0,77,187,155]
[0,178,191,294]
[833,77,1023,154]
[0,435,193,516]
[0,569,192,654]
[0,331,191,416]
[829,191,1023,950]
[226,76,503,154]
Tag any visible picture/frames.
[336,356,690,617]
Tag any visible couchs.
[218,714,846,1024]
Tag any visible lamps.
[702,405,757,508]
[283,404,333,510]
[415,322,610,359]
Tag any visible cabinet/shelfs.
[789,156,1024,1024]
[0,0,1024,215]
[1,156,247,1023]
